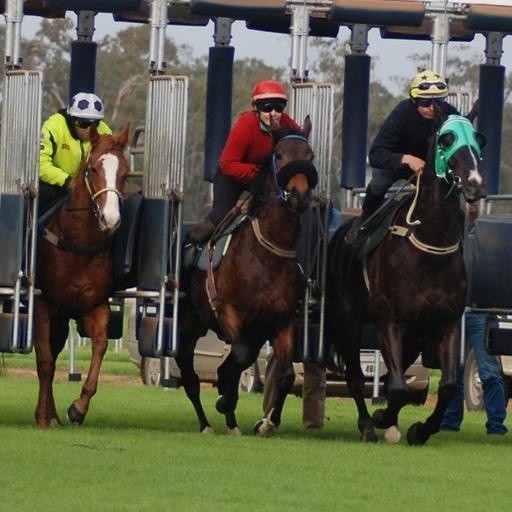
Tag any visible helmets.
[66,91,105,120]
[251,79,289,106]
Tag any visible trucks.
[419,194,512,414]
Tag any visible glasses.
[256,101,286,113]
[414,98,445,108]
[74,118,99,129]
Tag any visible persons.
[363,69,463,214]
[22,90,113,218]
[186,78,302,244]
[438,198,509,434]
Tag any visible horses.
[22,121,132,431]
[324,95,487,446]
[170,111,317,437]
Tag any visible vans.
[134,299,430,404]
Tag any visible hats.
[408,69,449,100]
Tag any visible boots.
[184,215,226,251]
[346,182,386,245]
